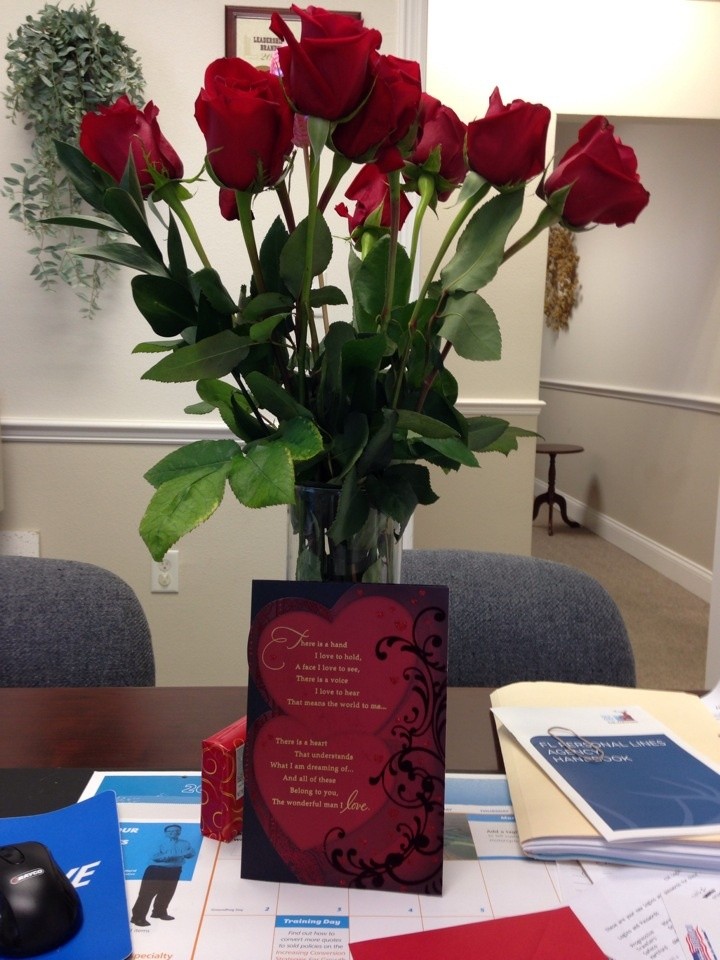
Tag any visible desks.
[0,686,720,960]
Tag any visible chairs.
[0,554,156,687]
[383,547,638,688]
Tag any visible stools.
[532,442,584,536]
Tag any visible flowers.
[37,3,651,581]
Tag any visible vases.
[279,471,401,583]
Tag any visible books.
[488,680,720,864]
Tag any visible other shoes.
[151,909,175,919]
[131,917,151,926]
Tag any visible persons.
[130,825,194,927]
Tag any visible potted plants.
[0,0,147,320]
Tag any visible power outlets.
[149,550,178,594]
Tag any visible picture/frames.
[224,4,362,75]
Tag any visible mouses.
[0,839,83,960]
